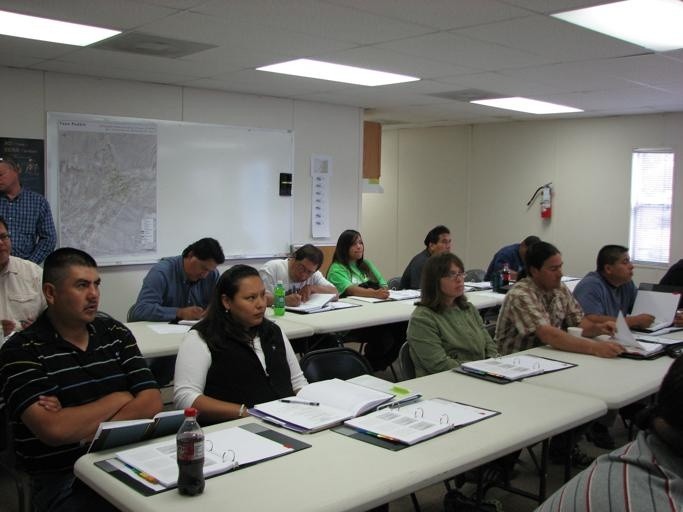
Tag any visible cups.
[567,327,583,337]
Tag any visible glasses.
[0,155,16,170]
[449,273,468,280]
[0,230,11,241]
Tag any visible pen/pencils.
[278,400,319,406]
[292,285,295,294]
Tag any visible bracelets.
[239,403,245,417]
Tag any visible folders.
[590,327,682,359]
[453,354,580,384]
[331,394,503,455]
[93,422,313,498]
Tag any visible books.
[285,292,337,312]
[560,275,579,282]
[461,357,544,380]
[463,281,514,293]
[178,319,201,326]
[114,438,233,489]
[628,289,682,333]
[345,406,452,446]
[247,376,396,435]
[347,287,422,303]
[87,407,197,453]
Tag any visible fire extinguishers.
[526,181,553,219]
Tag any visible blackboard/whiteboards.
[46,112,293,267]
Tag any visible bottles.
[177,408,205,496]
[274,280,285,316]
[502,263,510,286]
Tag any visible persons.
[573,245,682,430]
[398,225,452,290]
[0,153,57,265]
[493,242,628,469]
[325,230,406,372]
[483,235,541,280]
[406,251,504,512]
[131,237,225,321]
[0,247,164,511]
[534,351,682,512]
[0,212,48,348]
[172,264,310,418]
[259,243,339,306]
